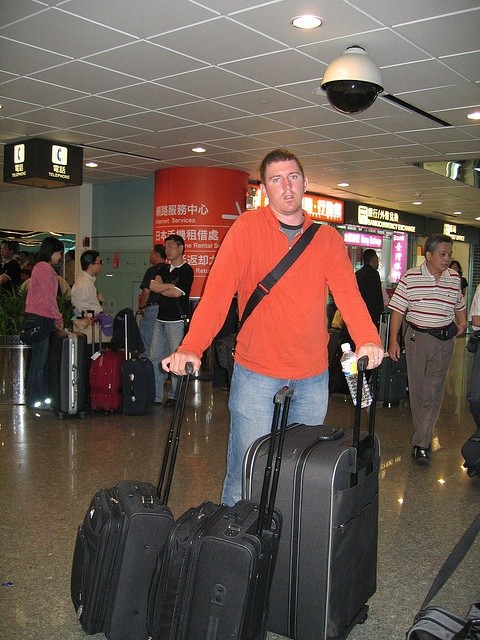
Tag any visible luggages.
[377,312,406,407]
[49,329,86,419]
[122,313,155,414]
[88,319,121,415]
[145,386,293,639]
[240,354,380,640]
[70,362,193,639]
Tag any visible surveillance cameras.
[320,46,384,115]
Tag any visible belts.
[75,316,81,318]
[146,303,156,305]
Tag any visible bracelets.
[460,320,467,331]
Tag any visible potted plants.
[0,283,34,406]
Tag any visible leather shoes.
[164,399,173,405]
[412,445,428,462]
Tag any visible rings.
[374,356,379,359]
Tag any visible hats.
[96,313,113,335]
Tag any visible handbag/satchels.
[216,223,321,380]
[405,515,479,639]
[409,322,457,340]
[465,330,478,352]
[174,297,190,333]
[19,315,54,343]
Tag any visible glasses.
[93,259,101,263]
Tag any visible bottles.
[339,342,373,409]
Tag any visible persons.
[448,260,468,297]
[0,240,21,295]
[149,234,194,407]
[388,234,467,466]
[161,148,384,518]
[59,250,75,286]
[140,244,167,359]
[17,276,71,299]
[355,249,384,333]
[26,237,64,409]
[69,250,104,343]
[459,283,480,429]
[19,250,41,282]
